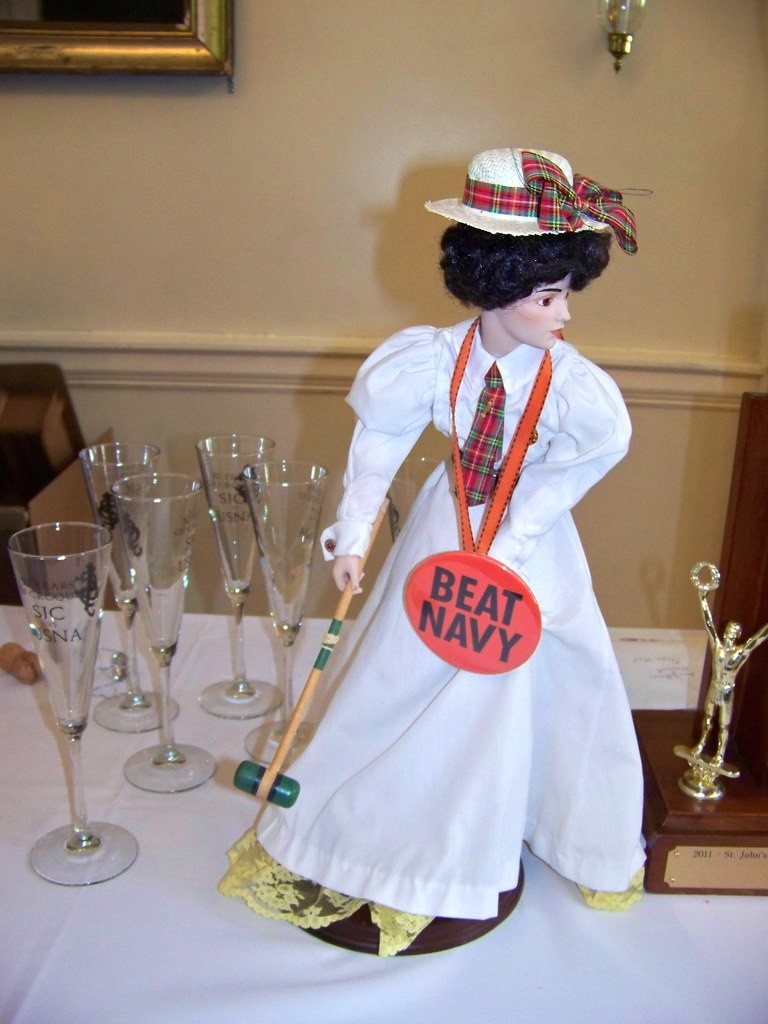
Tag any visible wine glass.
[8,522,139,887]
[111,471,218,792]
[194,434,283,720]
[242,460,328,771]
[78,441,179,734]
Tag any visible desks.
[0,605,768,1024]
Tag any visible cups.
[388,457,443,544]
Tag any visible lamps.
[599,0,648,74]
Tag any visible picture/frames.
[0,0,235,94]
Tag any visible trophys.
[673,560,768,802]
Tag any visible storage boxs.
[0,426,132,611]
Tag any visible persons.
[216,146,650,961]
